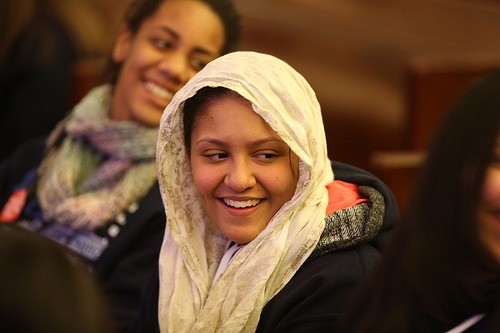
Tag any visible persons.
[334,68,500,333]
[0,0,241,333]
[102,50,402,333]
